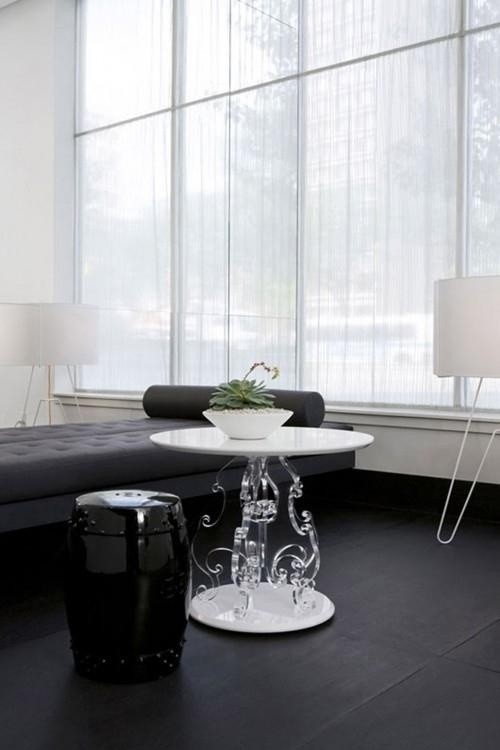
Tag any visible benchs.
[0,382,355,533]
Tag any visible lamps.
[0,301,106,429]
[431,277,500,545]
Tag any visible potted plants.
[203,360,296,442]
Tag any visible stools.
[65,485,194,687]
